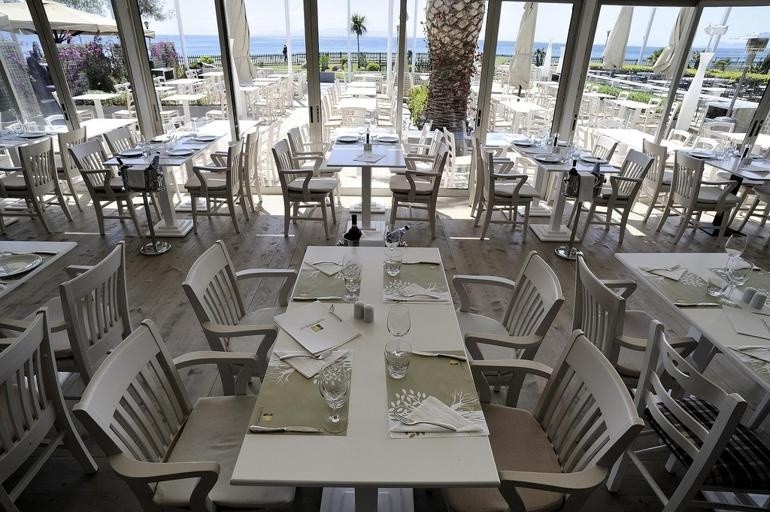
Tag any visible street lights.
[144,20,152,46]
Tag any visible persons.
[283,45,287,61]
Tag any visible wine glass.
[717,233,753,308]
[384,224,401,256]
[386,305,412,357]
[342,256,361,304]
[319,361,349,434]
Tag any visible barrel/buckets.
[592,171,605,197]
[146,167,163,190]
[565,174,580,195]
[342,232,360,246]
[119,166,128,187]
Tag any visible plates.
[688,151,764,159]
[118,133,217,158]
[338,135,399,143]
[513,140,607,165]
[0,253,43,277]
[18,132,46,139]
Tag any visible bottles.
[592,158,603,175]
[354,301,374,323]
[149,151,161,170]
[742,287,767,308]
[568,160,579,177]
[388,224,409,245]
[348,214,361,241]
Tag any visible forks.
[279,349,332,360]
[398,290,440,299]
[645,264,681,272]
[392,411,458,432]
[312,260,345,266]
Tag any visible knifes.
[293,297,342,300]
[248,424,320,434]
[401,261,440,265]
[674,300,720,308]
[410,348,467,362]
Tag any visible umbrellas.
[0,0,155,44]
[224,0,256,87]
[653,6,693,88]
[507,2,539,101]
[602,6,634,78]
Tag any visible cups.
[384,339,412,380]
[706,269,731,298]
[345,280,360,293]
[327,391,347,409]
[383,248,402,276]
